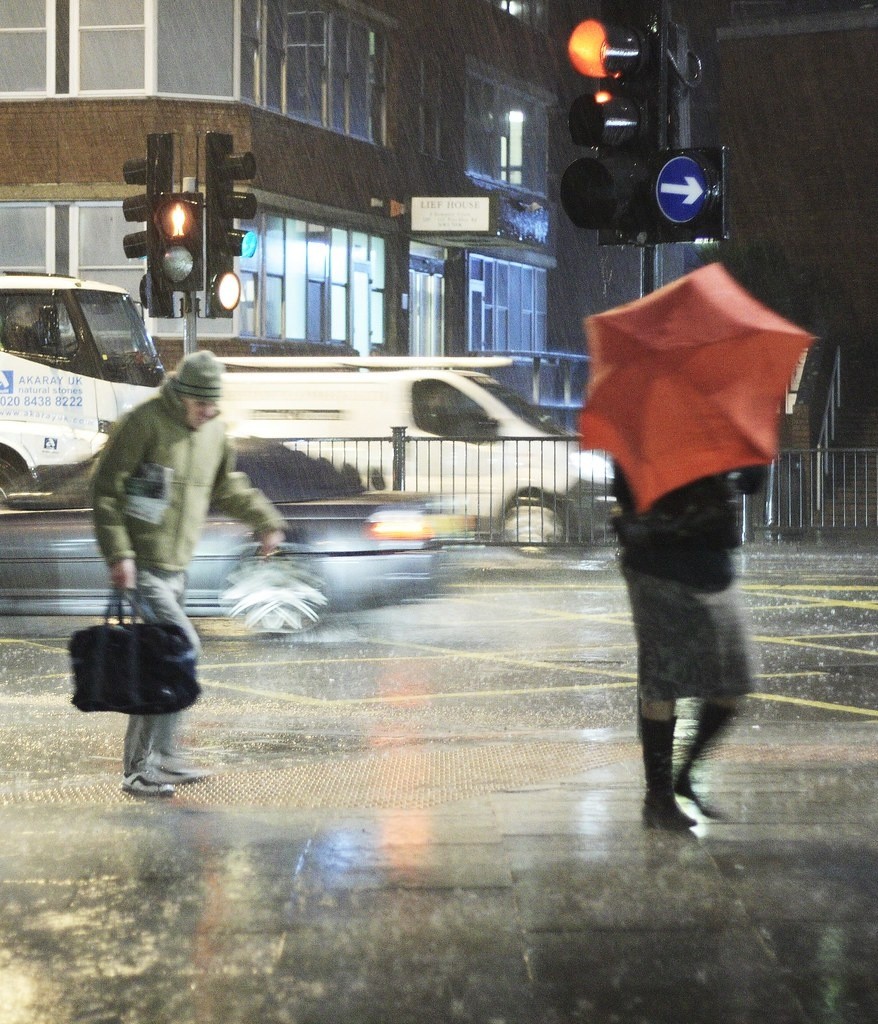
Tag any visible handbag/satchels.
[69,592,203,715]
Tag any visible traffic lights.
[559,0,667,247]
[200,129,258,319]
[121,133,183,319]
[152,191,204,292]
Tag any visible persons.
[93,347,287,802]
[612,450,773,829]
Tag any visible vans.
[189,348,619,557]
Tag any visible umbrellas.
[580,259,814,514]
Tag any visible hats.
[171,351,225,400]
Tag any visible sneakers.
[122,770,175,793]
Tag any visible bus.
[0,266,166,520]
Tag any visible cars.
[5,439,466,639]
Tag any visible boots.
[673,702,737,820]
[639,713,699,829]
